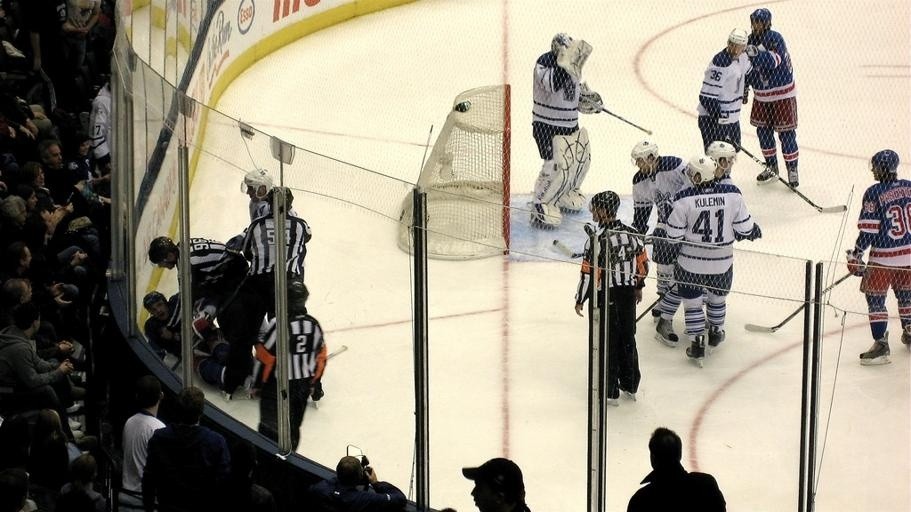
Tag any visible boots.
[685,333,705,359]
[708,324,725,346]
[785,158,799,188]
[859,328,890,358]
[900,323,911,344]
[756,153,779,181]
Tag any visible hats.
[336,455,363,489]
[461,458,525,494]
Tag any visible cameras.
[358,455,371,486]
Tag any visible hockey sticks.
[746,272,852,334]
[552,238,652,259]
[738,143,847,213]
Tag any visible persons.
[462,458,531,512]
[626,426,726,512]
[846,149,911,365]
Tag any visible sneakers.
[607,383,619,399]
[618,375,640,394]
[557,191,584,211]
[652,308,679,342]
[530,201,563,227]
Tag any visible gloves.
[746,43,760,58]
[843,249,866,277]
[191,305,219,343]
[309,380,324,401]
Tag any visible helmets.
[142,290,168,310]
[681,155,716,188]
[631,139,660,164]
[265,187,294,214]
[148,236,177,265]
[551,32,572,58]
[244,167,273,199]
[727,27,749,53]
[287,280,309,314]
[705,139,737,179]
[870,148,900,174]
[749,7,772,31]
[590,190,620,218]
